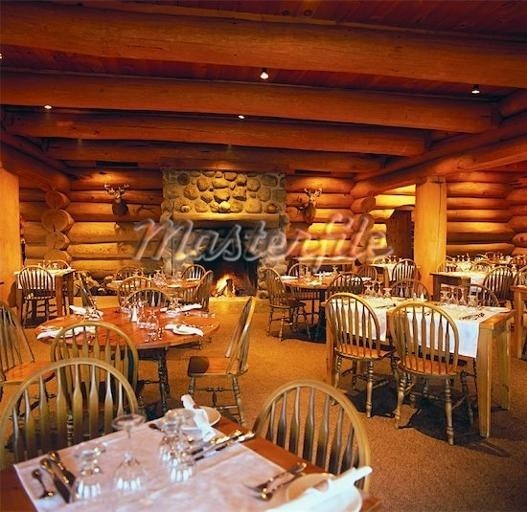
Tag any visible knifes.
[47,450,75,487]
[39,457,70,503]
[189,429,256,462]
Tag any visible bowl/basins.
[443,267,456,272]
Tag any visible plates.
[285,472,334,501]
[278,276,295,280]
[168,405,221,427]
[172,328,194,335]
[50,335,74,338]
[312,273,330,277]
[350,277,371,282]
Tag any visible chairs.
[326,270,517,446]
[263,247,527,341]
[1,357,385,512]
[0,258,256,431]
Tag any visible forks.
[243,461,306,500]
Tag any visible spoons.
[30,467,56,499]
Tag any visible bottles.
[452,255,471,273]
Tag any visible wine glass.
[157,408,196,497]
[439,285,476,314]
[111,412,151,510]
[135,303,158,330]
[120,268,197,288]
[364,280,391,301]
[70,440,111,512]
